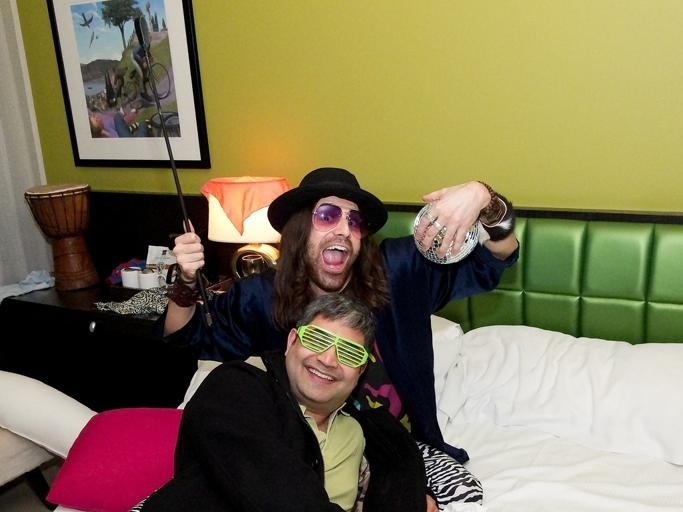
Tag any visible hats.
[267,166,388,236]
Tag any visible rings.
[423,212,435,222]
[433,221,442,231]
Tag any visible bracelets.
[478,180,516,243]
[165,275,201,306]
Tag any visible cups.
[119,266,168,292]
[240,254,265,278]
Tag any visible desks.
[0,282,198,411]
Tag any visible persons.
[139,292,440,510]
[127,167,520,511]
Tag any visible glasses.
[310,203,365,233]
[293,324,376,370]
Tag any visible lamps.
[201,176,296,278]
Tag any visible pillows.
[43,406,191,507]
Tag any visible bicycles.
[124,63,171,99]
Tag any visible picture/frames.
[43,0,213,171]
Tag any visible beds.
[51,198,683,512]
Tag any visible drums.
[24,183,100,292]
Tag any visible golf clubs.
[134,14,217,333]
